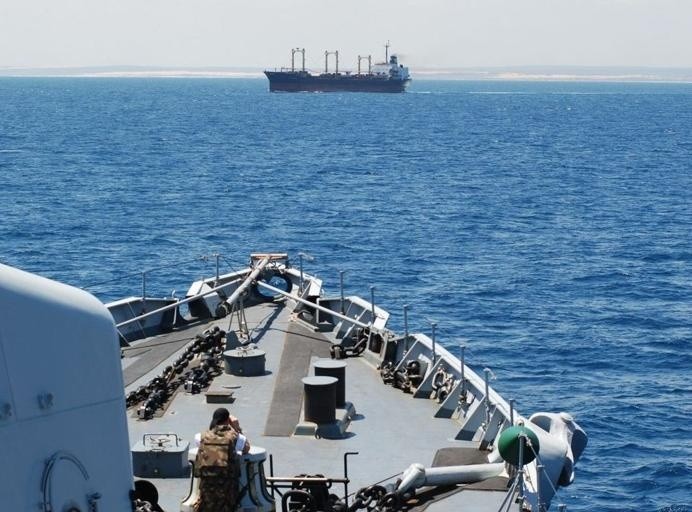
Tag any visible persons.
[193,407,251,511]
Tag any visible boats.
[1,252,588,508]
[262,39,412,93]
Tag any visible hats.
[209,407,230,431]
